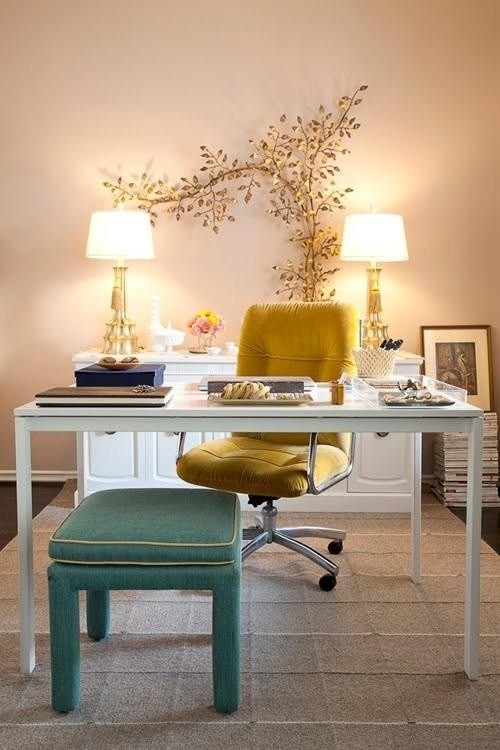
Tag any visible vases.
[190,335,211,354]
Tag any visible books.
[432,412,500,507]
[34,385,177,407]
[197,373,317,392]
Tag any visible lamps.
[339,204,409,349]
[84,203,156,354]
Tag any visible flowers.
[188,309,223,335]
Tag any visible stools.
[48,487,241,716]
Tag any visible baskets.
[352,345,396,378]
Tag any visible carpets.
[0,477,500,750]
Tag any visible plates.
[208,393,315,405]
[94,361,144,371]
[384,398,456,406]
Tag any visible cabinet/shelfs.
[72,351,423,513]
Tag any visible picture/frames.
[420,324,494,414]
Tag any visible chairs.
[178,301,359,590]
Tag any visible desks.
[13,379,484,679]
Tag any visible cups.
[204,341,239,356]
